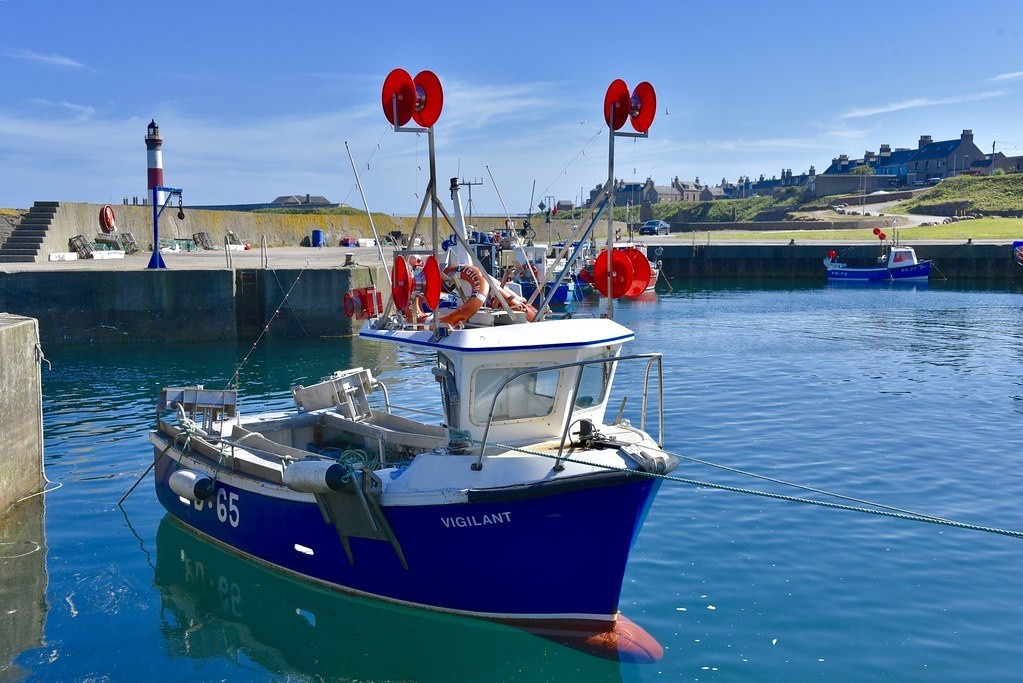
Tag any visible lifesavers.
[343,291,354,317]
[520,264,538,282]
[404,263,489,331]
[489,281,544,323]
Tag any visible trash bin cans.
[312,230,323,247]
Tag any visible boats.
[404,165,661,318]
[146,317,679,636]
[1012,241,1023,268]
[826,218,933,283]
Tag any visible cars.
[930,178,941,185]
[638,220,670,235]
